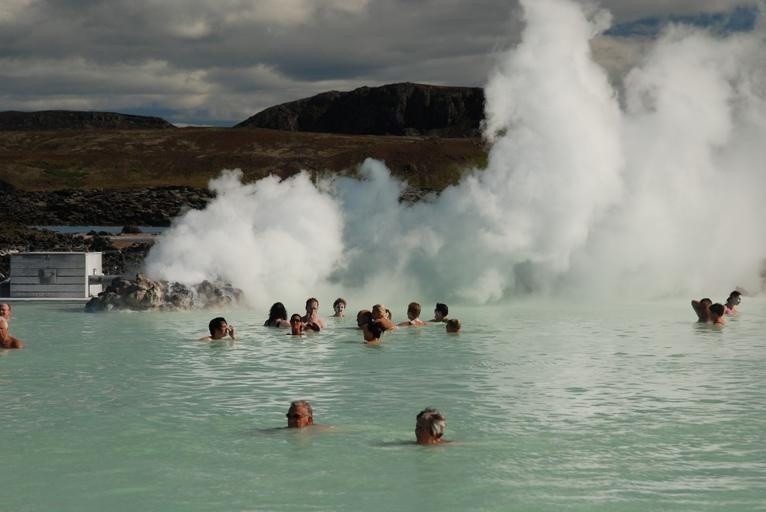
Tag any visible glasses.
[291,319,301,323]
[285,413,311,419]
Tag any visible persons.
[264,298,461,346]
[415,408,452,445]
[0,317,24,348]
[286,401,334,433]
[709,303,727,330]
[200,318,238,340]
[723,291,741,314]
[692,299,712,322]
[0,304,11,319]
